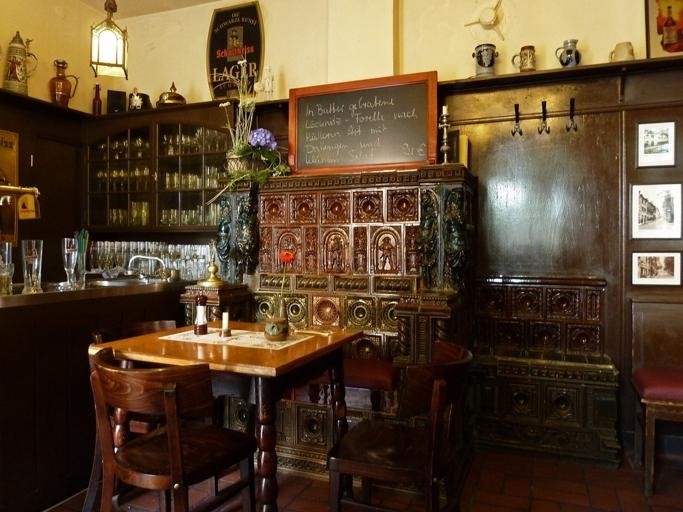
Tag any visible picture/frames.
[634,119,678,168]
[628,180,683,242]
[0,129,20,248]
[630,249,682,288]
[643,0,683,61]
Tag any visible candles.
[440,103,450,115]
[220,309,230,332]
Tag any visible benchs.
[177,272,460,493]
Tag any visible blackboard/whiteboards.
[288,71,438,176]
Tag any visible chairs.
[325,337,472,511]
[91,319,219,510]
[87,346,259,511]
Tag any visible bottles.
[193,294,208,334]
[92,83,102,117]
[656,8,664,36]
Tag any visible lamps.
[88,1,129,79]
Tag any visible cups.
[265,318,289,341]
[89,240,213,283]
[510,44,538,73]
[0,241,14,294]
[97,127,229,225]
[610,42,635,62]
[21,237,45,294]
[474,43,496,76]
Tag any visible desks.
[86,317,365,511]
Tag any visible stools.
[630,363,682,497]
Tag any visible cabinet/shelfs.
[84,98,239,232]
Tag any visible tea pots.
[48,58,79,108]
[554,38,582,66]
[3,30,39,98]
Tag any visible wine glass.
[59,236,78,286]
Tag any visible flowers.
[205,127,291,205]
[274,249,294,299]
[207,50,274,154]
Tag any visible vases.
[225,156,264,178]
[276,294,290,336]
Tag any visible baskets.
[225,151,262,173]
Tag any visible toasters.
[661,4,679,45]
